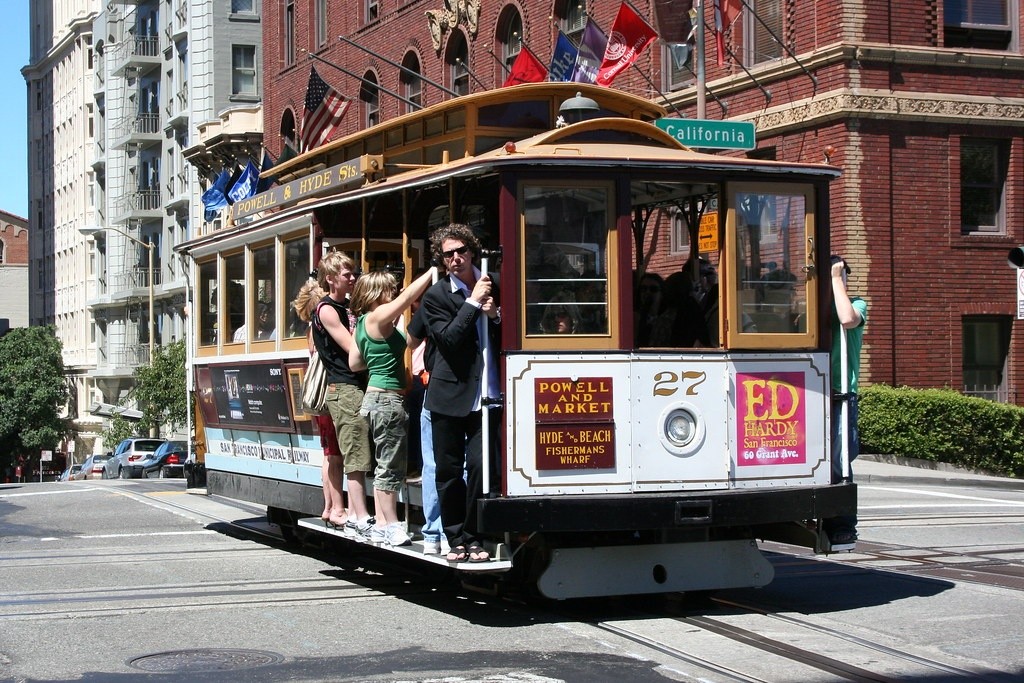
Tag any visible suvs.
[55,464,82,482]
[102,437,165,479]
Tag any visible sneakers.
[354,519,373,543]
[371,520,407,542]
[423,541,439,553]
[384,523,412,546]
[440,541,453,556]
[344,519,356,537]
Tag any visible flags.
[300,61,354,153]
[502,0,658,86]
[713,0,745,66]
[202,147,297,210]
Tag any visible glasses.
[443,245,469,259]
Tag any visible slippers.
[468,545,489,562]
[447,547,468,563]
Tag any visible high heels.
[329,519,343,530]
[322,517,330,527]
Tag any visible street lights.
[79,226,157,440]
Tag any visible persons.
[755,270,806,333]
[295,251,451,555]
[799,256,867,482]
[419,223,502,563]
[525,218,719,347]
[211,282,307,344]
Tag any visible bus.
[172,89,858,605]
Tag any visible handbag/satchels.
[301,351,330,415]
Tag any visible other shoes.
[831,530,856,545]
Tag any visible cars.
[143,439,188,479]
[74,455,113,481]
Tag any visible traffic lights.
[1008,247,1024,269]
